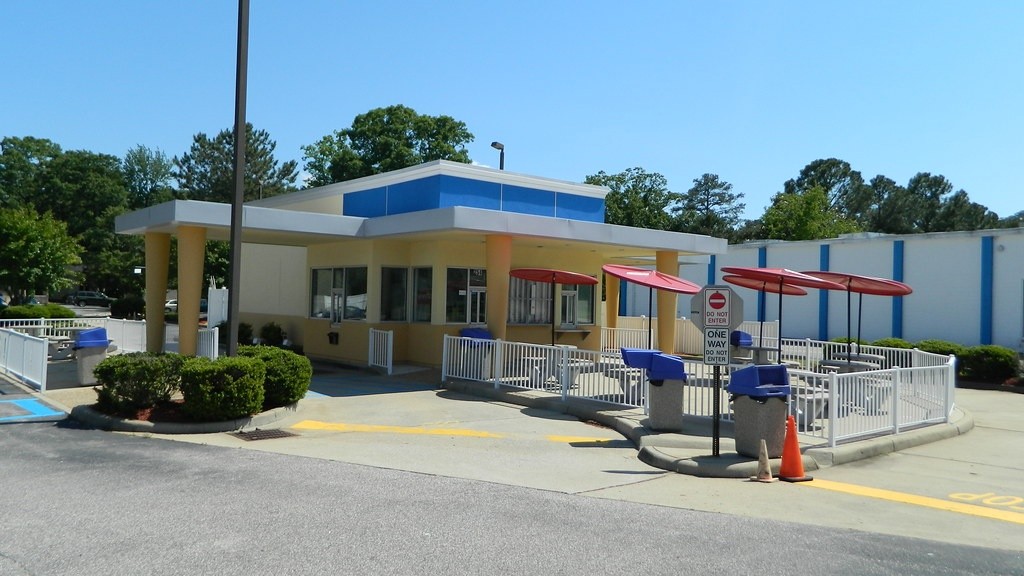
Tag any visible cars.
[164,299,177,313]
[315,306,367,320]
[202,299,208,312]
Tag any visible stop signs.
[705,286,728,327]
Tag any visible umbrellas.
[722,274,808,349]
[509,269,596,346]
[720,265,845,364]
[800,270,912,362]
[603,264,702,349]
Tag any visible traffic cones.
[751,439,777,483]
[778,415,813,482]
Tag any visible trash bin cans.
[730,331,753,364]
[72,327,110,386]
[723,365,791,459]
[621,347,688,432]
[460,328,492,379]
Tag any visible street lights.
[491,142,504,170]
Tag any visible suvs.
[75,290,119,308]
